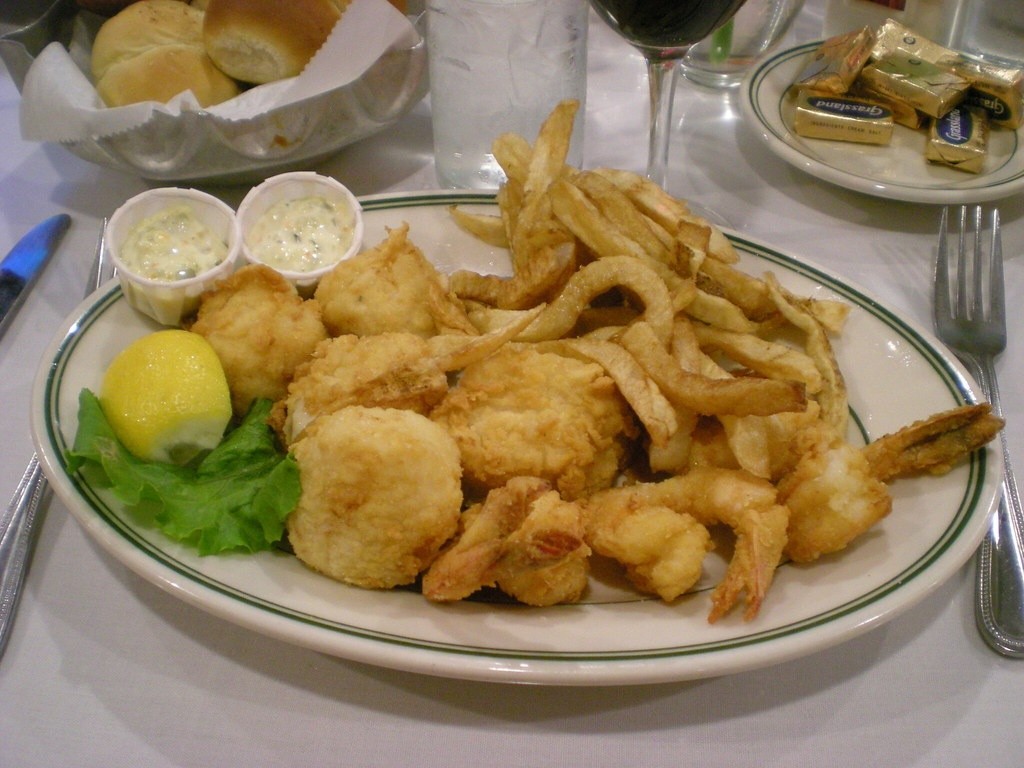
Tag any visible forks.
[0,216,118,662]
[931,202,1024,659]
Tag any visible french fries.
[447,99,846,471]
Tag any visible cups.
[680,0,804,89]
[426,0,587,189]
[904,0,1024,70]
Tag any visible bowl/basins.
[237,171,366,299]
[107,186,241,332]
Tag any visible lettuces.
[64,388,304,556]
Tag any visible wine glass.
[587,0,746,231]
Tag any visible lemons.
[100,330,233,464]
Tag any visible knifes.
[0,213,71,346]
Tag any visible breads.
[90,0,409,109]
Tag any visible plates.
[32,189,1004,687]
[739,36,1024,205]
